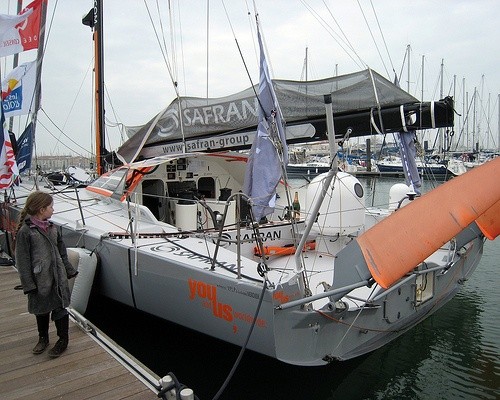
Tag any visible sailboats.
[0,0,500,367]
[377,44,499,175]
[288,140,344,174]
[344,136,381,176]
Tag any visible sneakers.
[33,336,49,353]
[48,338,68,356]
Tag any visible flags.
[0,0,48,58]
[242,20,289,224]
[393,76,422,196]
[2,60,41,117]
[0,119,34,191]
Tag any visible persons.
[460,152,474,162]
[87,162,95,180]
[11,192,79,357]
